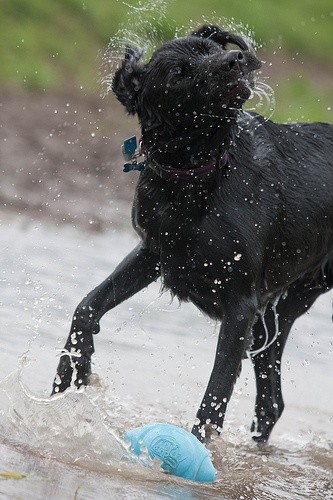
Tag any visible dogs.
[49,25,333,447]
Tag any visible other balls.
[123,424,218,482]
